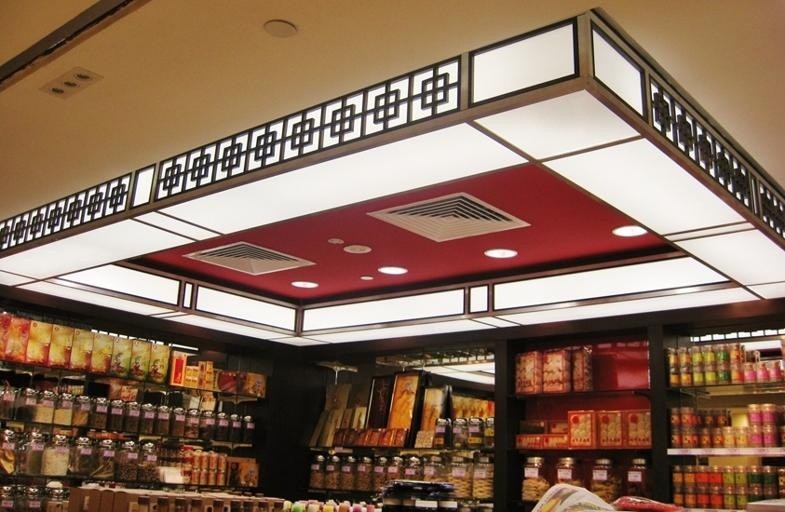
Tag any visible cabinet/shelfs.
[300,362,495,511]
[496,323,785,511]
[2,362,267,512]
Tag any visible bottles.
[136,495,284,512]
[0,425,160,484]
[309,453,494,512]
[0,480,68,512]
[520,454,651,504]
[433,415,495,451]
[0,380,257,446]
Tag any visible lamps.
[0,12,784,347]
